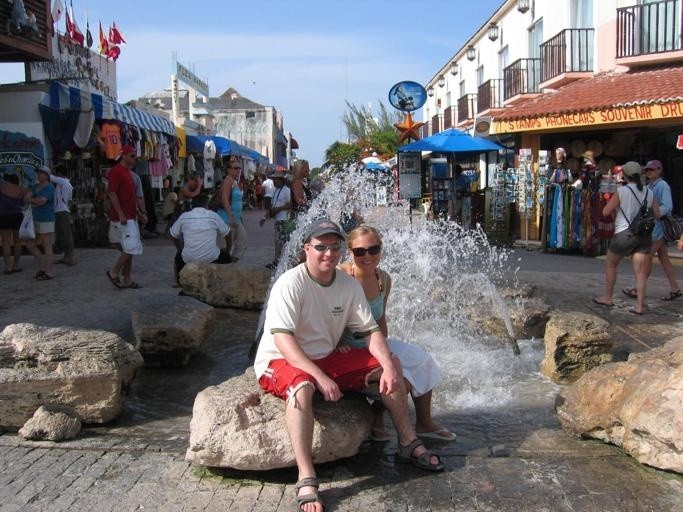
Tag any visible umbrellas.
[398,128,505,192]
[366,163,384,170]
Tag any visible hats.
[115,145,136,161]
[303,219,345,245]
[644,160,661,171]
[38,166,51,175]
[622,161,642,178]
[267,171,286,179]
[580,150,595,162]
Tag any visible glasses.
[352,244,381,257]
[308,243,342,251]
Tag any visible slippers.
[107,270,124,288]
[590,295,616,306]
[33,270,53,281]
[5,268,22,275]
[628,305,644,315]
[124,280,141,289]
[368,426,395,441]
[415,426,457,442]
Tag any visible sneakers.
[265,261,277,272]
[55,258,75,265]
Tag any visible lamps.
[466,45,475,62]
[438,78,444,87]
[487,23,498,41]
[517,0,529,15]
[449,62,458,76]
[428,86,434,97]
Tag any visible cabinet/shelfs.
[431,177,453,217]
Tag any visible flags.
[52,0,127,62]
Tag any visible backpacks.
[618,185,656,236]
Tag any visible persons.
[550,147,574,183]
[254,220,445,512]
[393,84,411,110]
[451,164,471,229]
[0,165,77,280]
[591,161,661,315]
[334,226,456,441]
[572,150,598,190]
[170,195,232,287]
[621,159,683,301]
[107,159,312,277]
[106,145,148,288]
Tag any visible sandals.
[293,476,326,512]
[397,438,446,471]
[659,287,683,301]
[621,286,639,298]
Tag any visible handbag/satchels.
[216,189,231,208]
[18,205,35,240]
[661,214,682,243]
